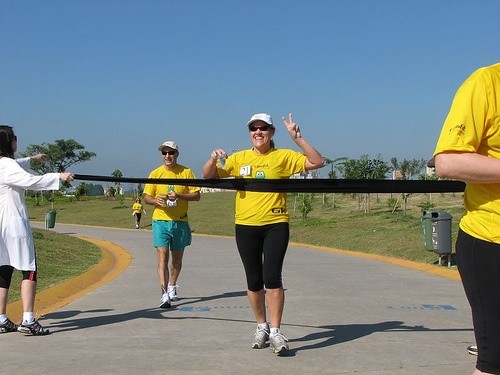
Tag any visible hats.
[247,113,272,128]
[158,141,179,154]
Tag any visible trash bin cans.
[46,211,57,228]
[422,209,452,255]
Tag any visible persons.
[0,125,76,336]
[202,113,325,356]
[132,197,147,229]
[432,63,500,375]
[142,142,201,308]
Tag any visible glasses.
[162,151,178,155]
[250,126,271,131]
[14,136,17,141]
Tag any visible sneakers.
[17,321,49,336]
[167,285,178,301]
[0,318,18,332]
[252,324,270,349]
[467,345,479,355]
[269,333,289,355]
[159,293,172,308]
